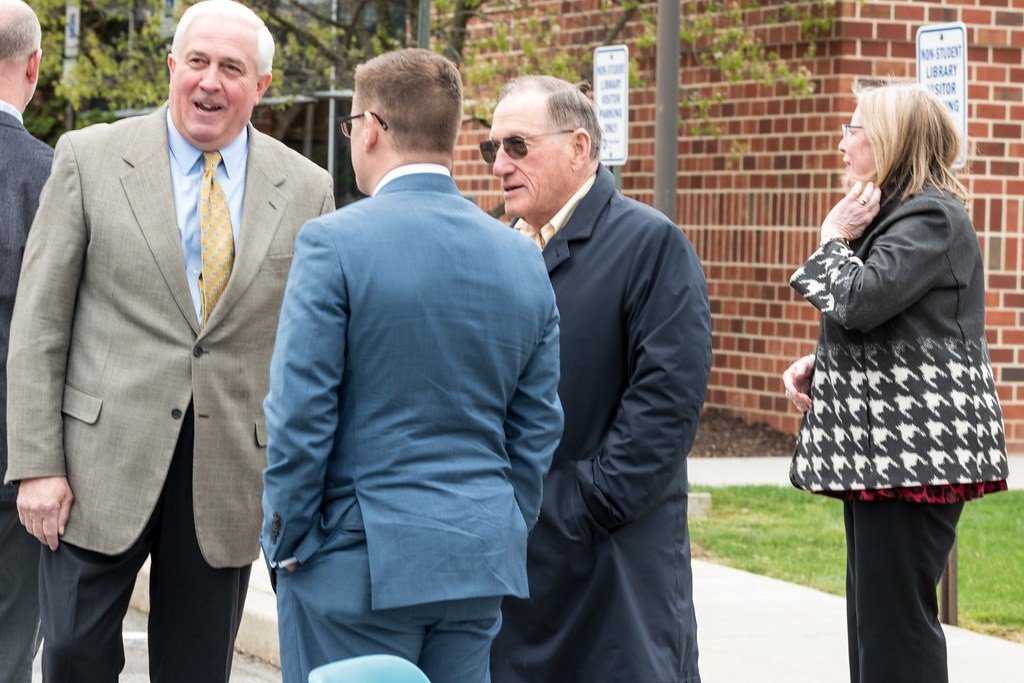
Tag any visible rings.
[785,389,789,398]
[856,197,866,205]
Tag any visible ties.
[199,151,235,330]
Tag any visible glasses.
[338,112,388,139]
[842,123,863,141]
[479,130,576,165]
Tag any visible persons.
[782,70,1010,683]
[4,0,337,683]
[259,49,562,682]
[0,0,56,683]
[478,73,710,683]
